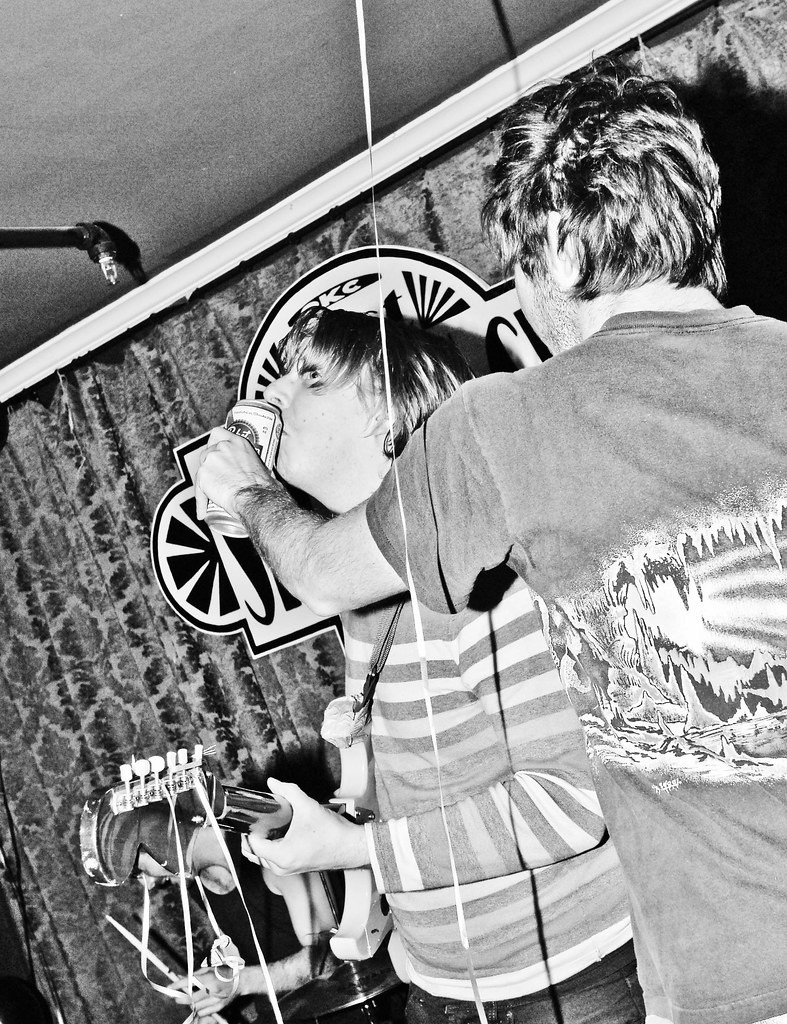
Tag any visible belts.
[411,940,636,1023]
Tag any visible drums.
[78,785,241,896]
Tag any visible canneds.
[202,399,283,539]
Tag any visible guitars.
[104,744,395,963]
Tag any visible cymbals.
[267,955,403,1024]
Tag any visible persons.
[81,782,407,1024]
[194,62,787,1024]
[241,306,646,1024]
